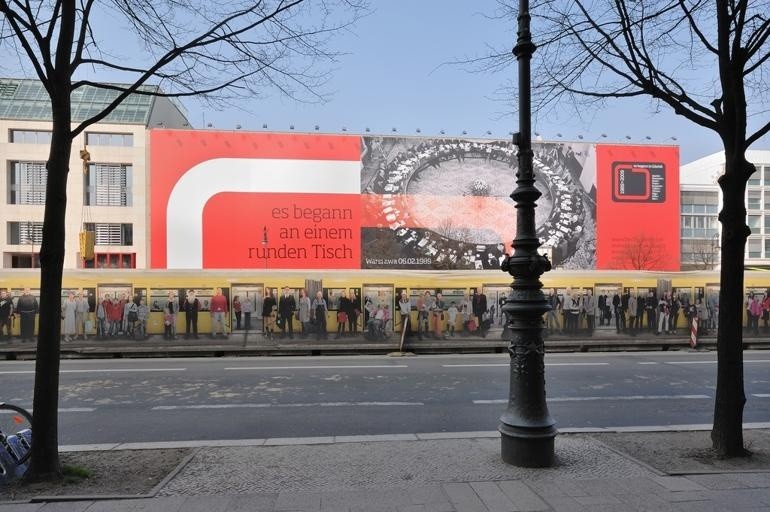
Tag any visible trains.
[1,269,770,338]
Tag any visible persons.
[376,140,587,268]
[0,286,39,342]
[60,288,252,343]
[546,288,717,338]
[745,290,770,335]
[259,286,507,337]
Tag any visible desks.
[364,137,585,270]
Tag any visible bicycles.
[0,401,33,465]
